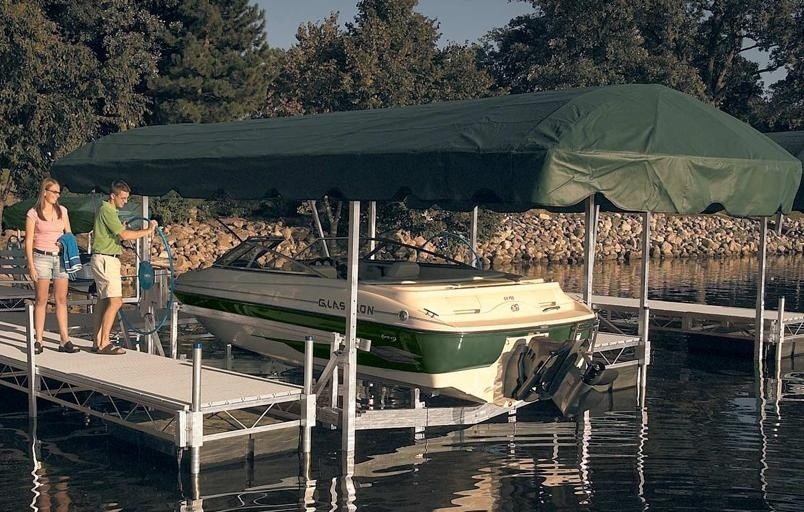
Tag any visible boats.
[168,232,619,419]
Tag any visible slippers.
[91,344,126,355]
[33,341,43,354]
[57,341,79,353]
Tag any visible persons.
[89,179,159,355]
[25,177,81,354]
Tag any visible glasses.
[45,189,60,194]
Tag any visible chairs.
[359,260,420,281]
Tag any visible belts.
[32,247,61,256]
[91,251,118,258]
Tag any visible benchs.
[0,249,36,311]
